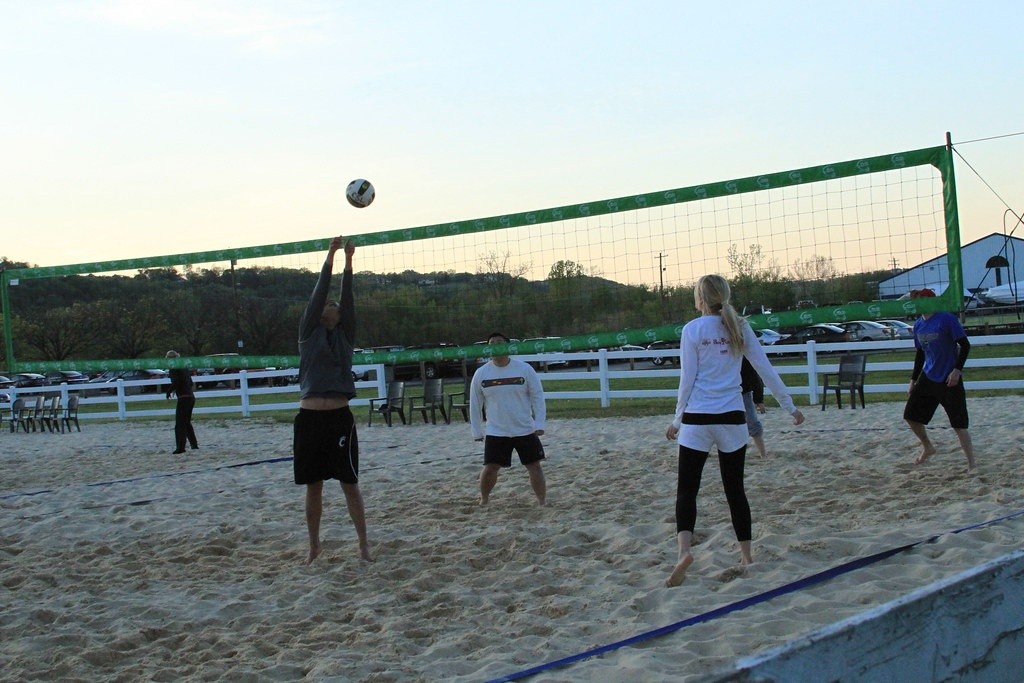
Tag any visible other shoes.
[191,446,199,449]
[173,450,186,454]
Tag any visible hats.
[911,289,935,297]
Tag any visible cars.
[837,320,902,342]
[753,328,791,345]
[0,336,644,399]
[815,322,841,327]
[770,324,845,357]
[875,320,915,340]
[962,287,992,314]
[645,339,680,365]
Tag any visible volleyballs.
[345,179,376,208]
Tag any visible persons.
[902,288,975,473]
[469,333,546,504]
[165,351,199,454]
[664,275,805,588]
[740,355,766,458]
[293,235,371,562]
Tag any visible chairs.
[56,395,80,434]
[33,396,61,433]
[368,382,406,429]
[16,397,45,433]
[447,374,487,426]
[1,398,26,433]
[821,354,867,410]
[408,379,447,427]
